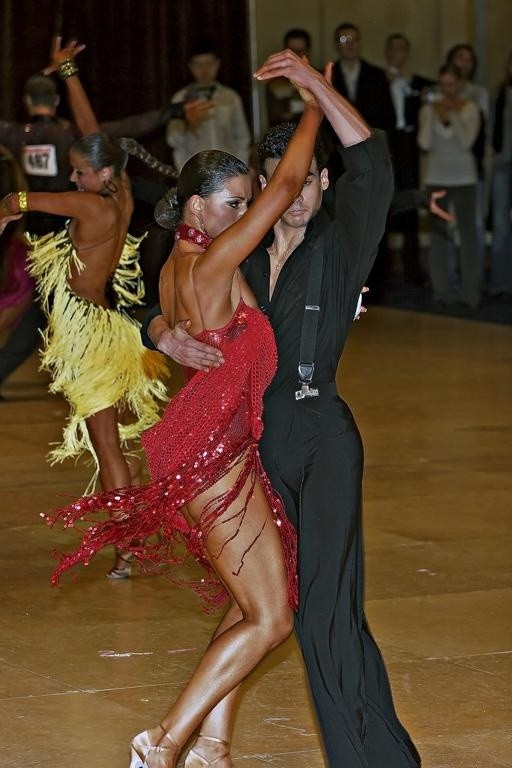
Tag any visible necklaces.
[271,242,298,270]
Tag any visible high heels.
[105,538,148,579]
[129,724,237,768]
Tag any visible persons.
[1,74,215,384]
[328,23,397,156]
[40,48,424,767]
[166,48,253,173]
[130,54,334,767]
[1,34,172,579]
[379,32,512,317]
[264,26,324,126]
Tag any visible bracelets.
[58,58,78,81]
